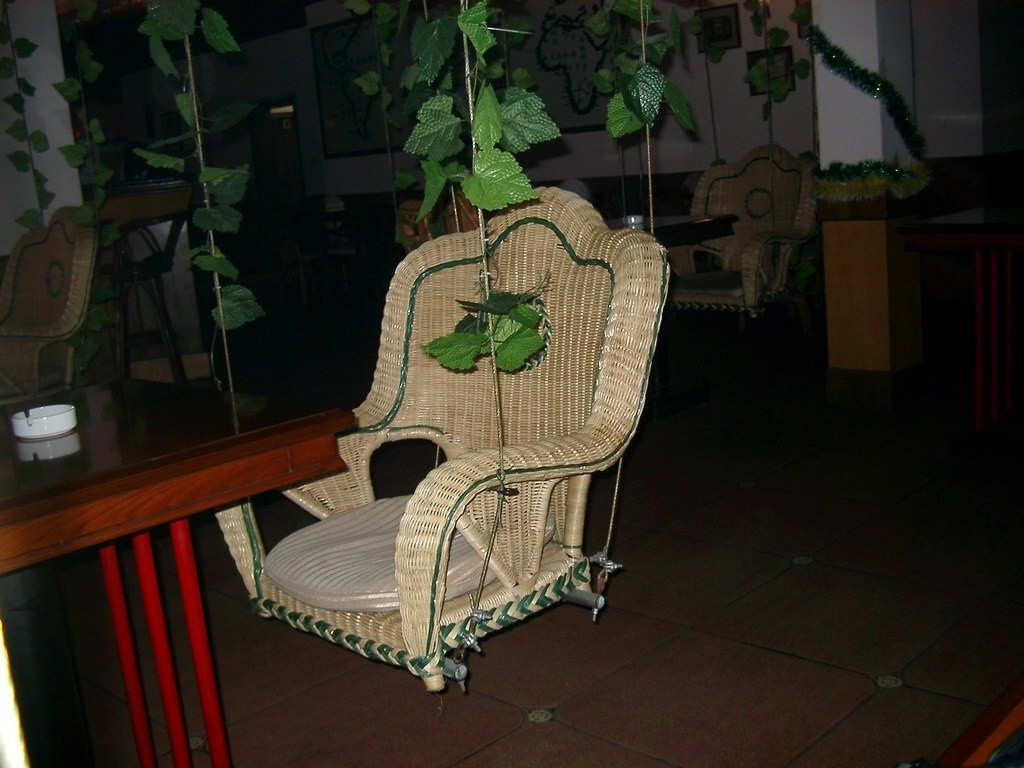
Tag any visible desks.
[609,215,743,424]
[896,207,1023,431]
[0,370,361,768]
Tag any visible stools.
[97,211,188,386]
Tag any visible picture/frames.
[308,0,606,158]
[694,3,742,52]
[745,45,796,95]
